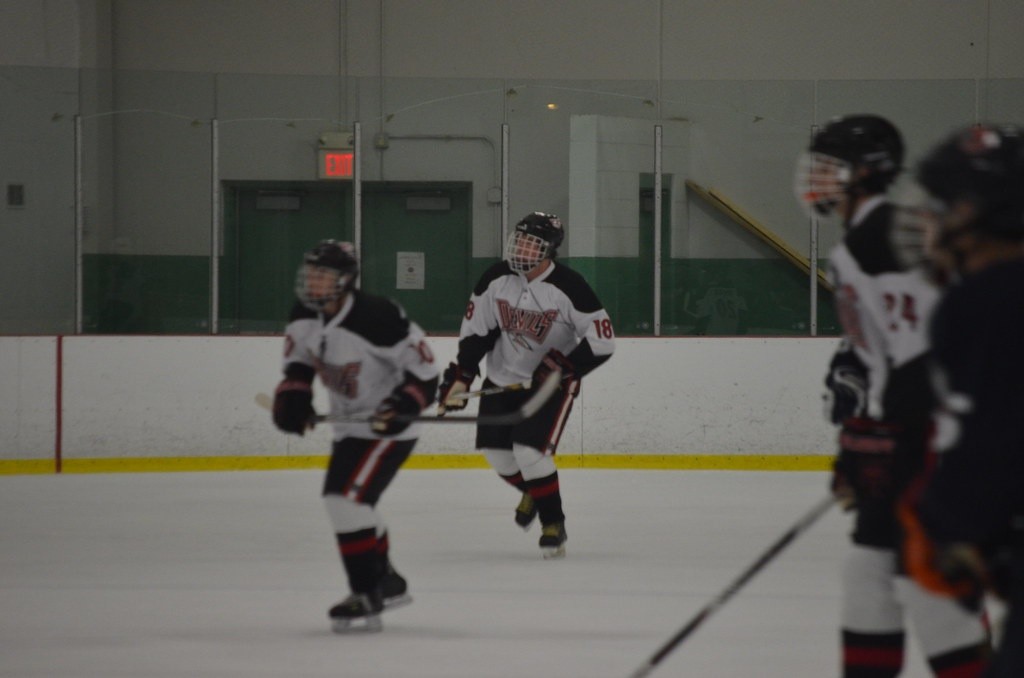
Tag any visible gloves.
[439,362,475,411]
[369,386,426,436]
[821,342,873,421]
[531,348,570,398]
[274,364,314,436]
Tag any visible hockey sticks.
[252,368,564,428]
[253,376,531,429]
[630,494,841,678]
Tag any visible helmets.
[504,212,564,273]
[295,240,357,311]
[916,124,1024,260]
[796,113,905,219]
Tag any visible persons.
[795,112,1024,678]
[438,212,615,559]
[272,237,439,634]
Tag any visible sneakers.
[539,519,566,558]
[378,574,413,610]
[329,590,383,632]
[516,493,537,532]
